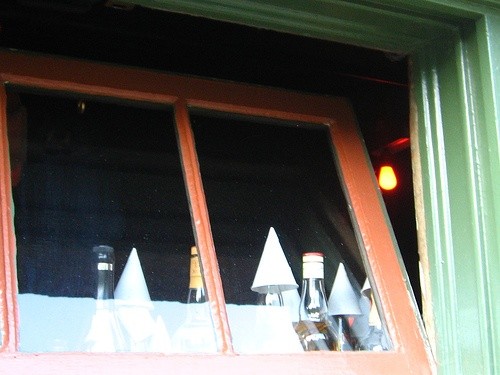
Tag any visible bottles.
[90,244,116,302]
[298,250,331,325]
[186,243,209,304]
